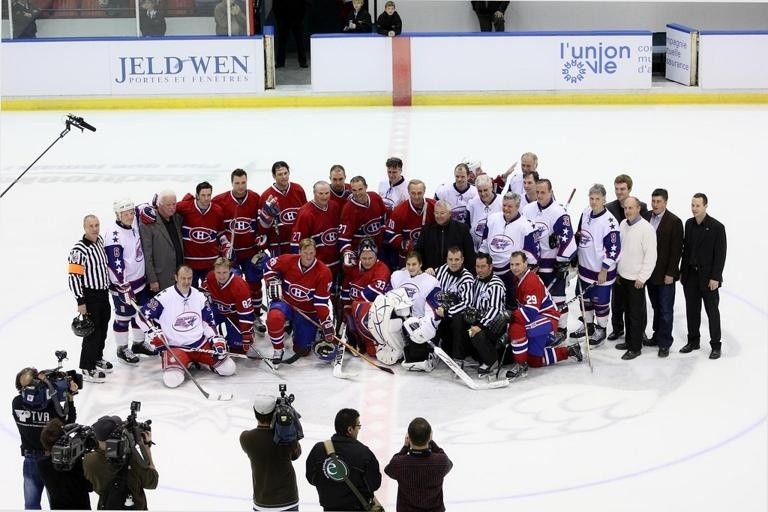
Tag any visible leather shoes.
[643,337,658,346]
[709,349,720,359]
[622,349,640,360]
[658,346,669,357]
[615,343,628,350]
[607,331,624,340]
[679,344,700,353]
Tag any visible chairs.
[32,0,195,17]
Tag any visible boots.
[570,316,594,338]
[566,343,583,361]
[132,341,158,355]
[117,345,139,363]
[551,328,567,346]
[253,313,266,332]
[589,323,606,345]
[506,361,528,377]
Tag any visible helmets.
[464,307,481,323]
[113,198,136,221]
[434,291,460,308]
[463,159,481,175]
[72,317,94,337]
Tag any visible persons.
[679,192,727,359]
[603,175,651,344]
[271,3,309,67]
[12,2,40,39]
[83,415,158,510]
[214,1,246,36]
[68,203,155,384]
[140,1,165,37]
[383,417,453,511]
[135,153,584,387]
[470,1,510,32]
[642,186,684,357]
[12,367,81,509]
[306,408,382,511]
[613,197,658,361]
[36,418,93,510]
[101,2,130,18]
[342,0,403,37]
[570,183,622,347]
[239,395,301,511]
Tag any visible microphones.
[65,112,97,132]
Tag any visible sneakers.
[272,349,284,363]
[478,359,497,374]
[82,369,105,378]
[452,359,464,373]
[96,359,113,369]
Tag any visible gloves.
[219,235,236,263]
[552,259,571,282]
[144,327,168,349]
[138,203,157,224]
[258,195,280,227]
[115,281,138,305]
[401,239,417,252]
[241,331,254,351]
[343,249,357,268]
[207,335,229,360]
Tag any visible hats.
[357,237,378,258]
[254,393,276,414]
[94,416,122,440]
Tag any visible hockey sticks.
[168,345,308,364]
[577,278,594,374]
[333,322,360,378]
[281,297,394,375]
[496,277,557,379]
[129,298,236,401]
[226,318,284,379]
[404,316,509,390]
